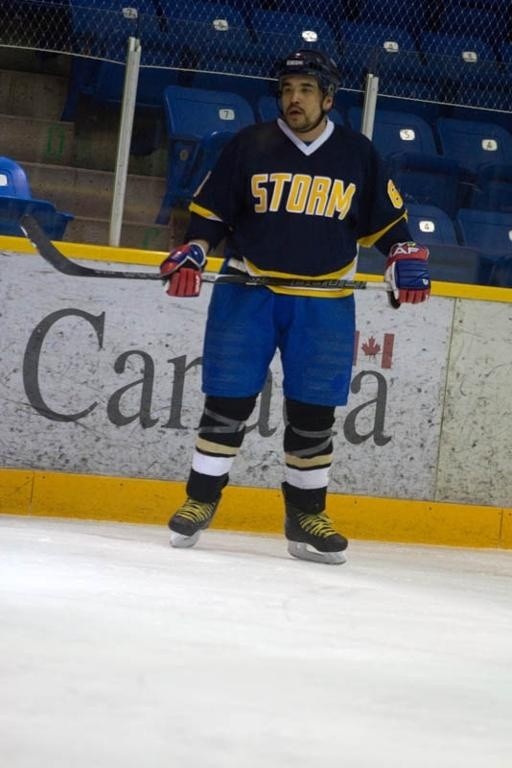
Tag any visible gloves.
[383,241,431,304]
[161,243,207,297]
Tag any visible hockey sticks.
[19,215,393,291]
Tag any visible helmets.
[277,49,343,95]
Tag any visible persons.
[159,50,432,550]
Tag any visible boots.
[280,483,347,552]
[168,476,229,535]
